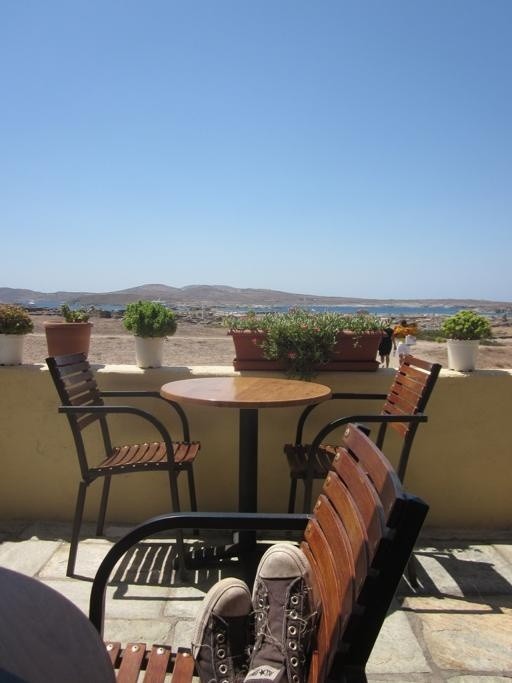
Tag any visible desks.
[0,566,117,683]
[160,377,332,512]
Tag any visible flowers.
[227,306,396,382]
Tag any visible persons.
[378,321,397,368]
[188,540,324,683]
[393,319,417,371]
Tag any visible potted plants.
[0,300,177,368]
[445,308,494,371]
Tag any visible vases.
[228,330,386,370]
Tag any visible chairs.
[283,353,443,510]
[45,354,203,576]
[88,425,431,683]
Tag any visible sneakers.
[193,575,252,683]
[245,541,323,683]
